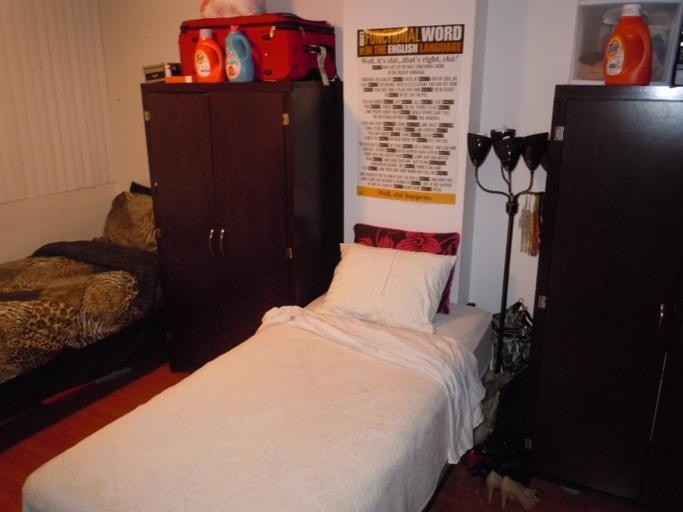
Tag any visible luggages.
[178,14,336,82]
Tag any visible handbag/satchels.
[492,302,533,376]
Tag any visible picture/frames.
[569,0,683,84]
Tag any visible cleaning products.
[193,29,223,82]
[603,5,650,85]
[223,23,254,82]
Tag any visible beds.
[22,289,495,512]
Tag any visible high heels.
[486,470,542,511]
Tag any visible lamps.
[468,128,551,369]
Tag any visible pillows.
[321,246,459,335]
[354,224,459,314]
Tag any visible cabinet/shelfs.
[140,82,344,372]
[520,84,683,512]
